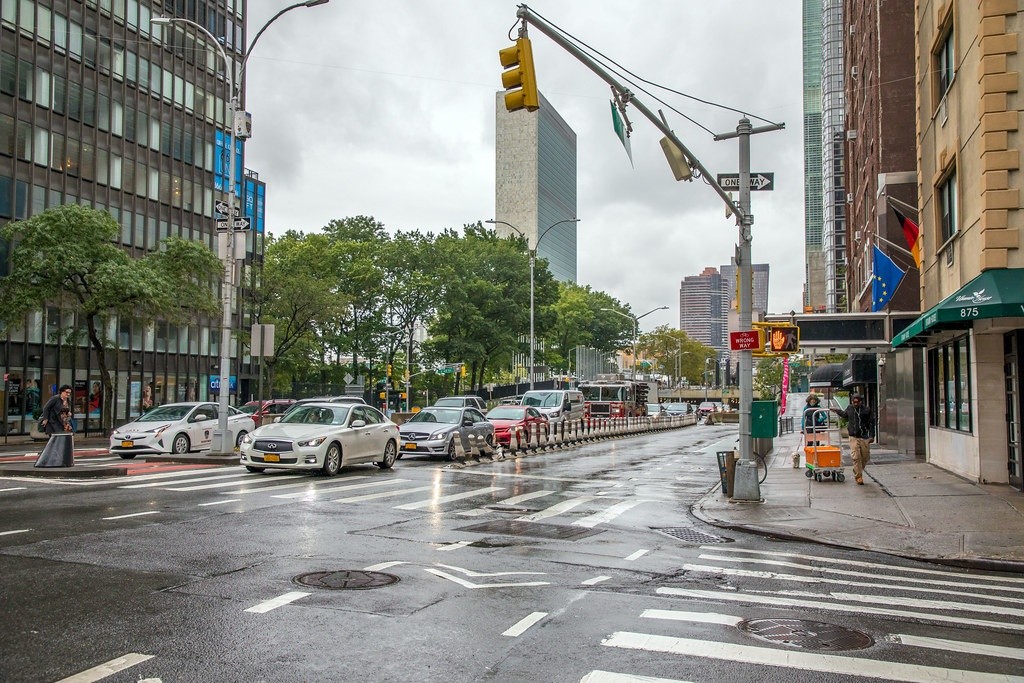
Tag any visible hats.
[806,393,820,405]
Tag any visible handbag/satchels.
[37,413,46,432]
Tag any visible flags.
[892,205,920,269]
[872,242,905,312]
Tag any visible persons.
[144,385,153,409]
[41,384,74,434]
[90,382,100,411]
[830,396,875,485]
[800,394,827,446]
[311,411,322,421]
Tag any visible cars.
[109,402,255,459]
[647,402,731,422]
[396,407,495,462]
[240,402,401,476]
[486,405,550,446]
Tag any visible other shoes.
[856,475,863,485]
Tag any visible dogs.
[791,452,801,468]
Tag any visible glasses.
[65,390,71,394]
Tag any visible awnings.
[892,268,1024,348]
[810,354,877,387]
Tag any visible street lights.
[655,350,690,390]
[485,218,581,390]
[569,344,615,378]
[707,357,737,389]
[150,0,330,455]
[600,306,669,383]
[664,334,681,403]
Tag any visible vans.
[515,390,584,436]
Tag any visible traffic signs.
[717,172,774,191]
[215,199,251,233]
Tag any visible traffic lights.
[405,370,410,381]
[380,393,385,399]
[387,364,391,376]
[771,326,800,352]
[400,393,406,398]
[499,38,539,113]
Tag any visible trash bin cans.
[716,451,740,497]
[781,417,794,434]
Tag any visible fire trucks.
[578,379,651,429]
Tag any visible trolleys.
[803,408,845,482]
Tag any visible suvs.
[434,395,488,415]
[237,395,367,430]
